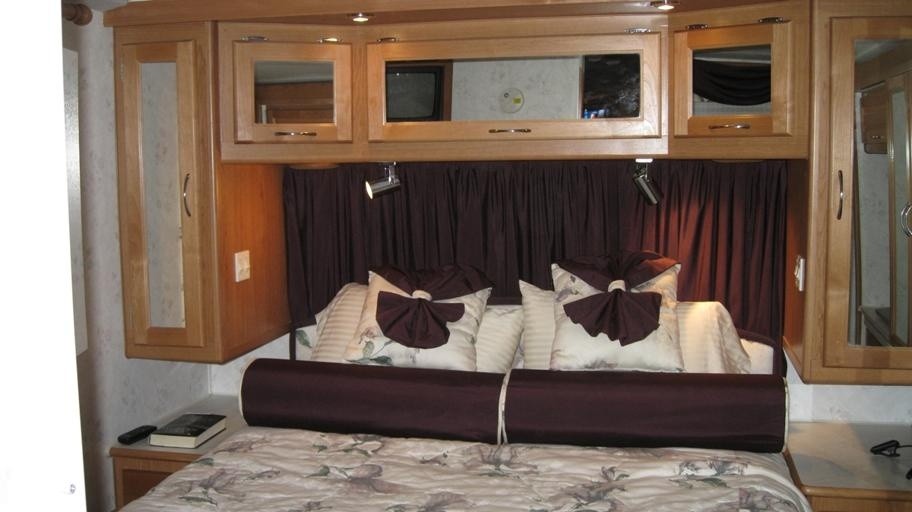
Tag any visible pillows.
[508,277,755,378]
[503,362,791,458]
[551,258,686,371]
[350,266,494,371]
[312,279,523,375]
[234,353,507,448]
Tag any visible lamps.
[357,158,403,199]
[629,155,662,208]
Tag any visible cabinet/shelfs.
[210,2,814,164]
[779,0,912,388]
[103,1,293,365]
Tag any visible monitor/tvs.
[386,65,444,121]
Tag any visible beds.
[99,279,819,512]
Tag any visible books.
[148,414,226,449]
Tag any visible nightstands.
[779,420,912,512]
[105,394,242,510]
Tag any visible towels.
[379,266,495,348]
[559,247,682,345]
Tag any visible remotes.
[118,425,157,445]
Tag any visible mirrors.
[251,58,336,126]
[131,57,188,331]
[383,51,641,122]
[689,42,772,121]
[849,27,910,351]
